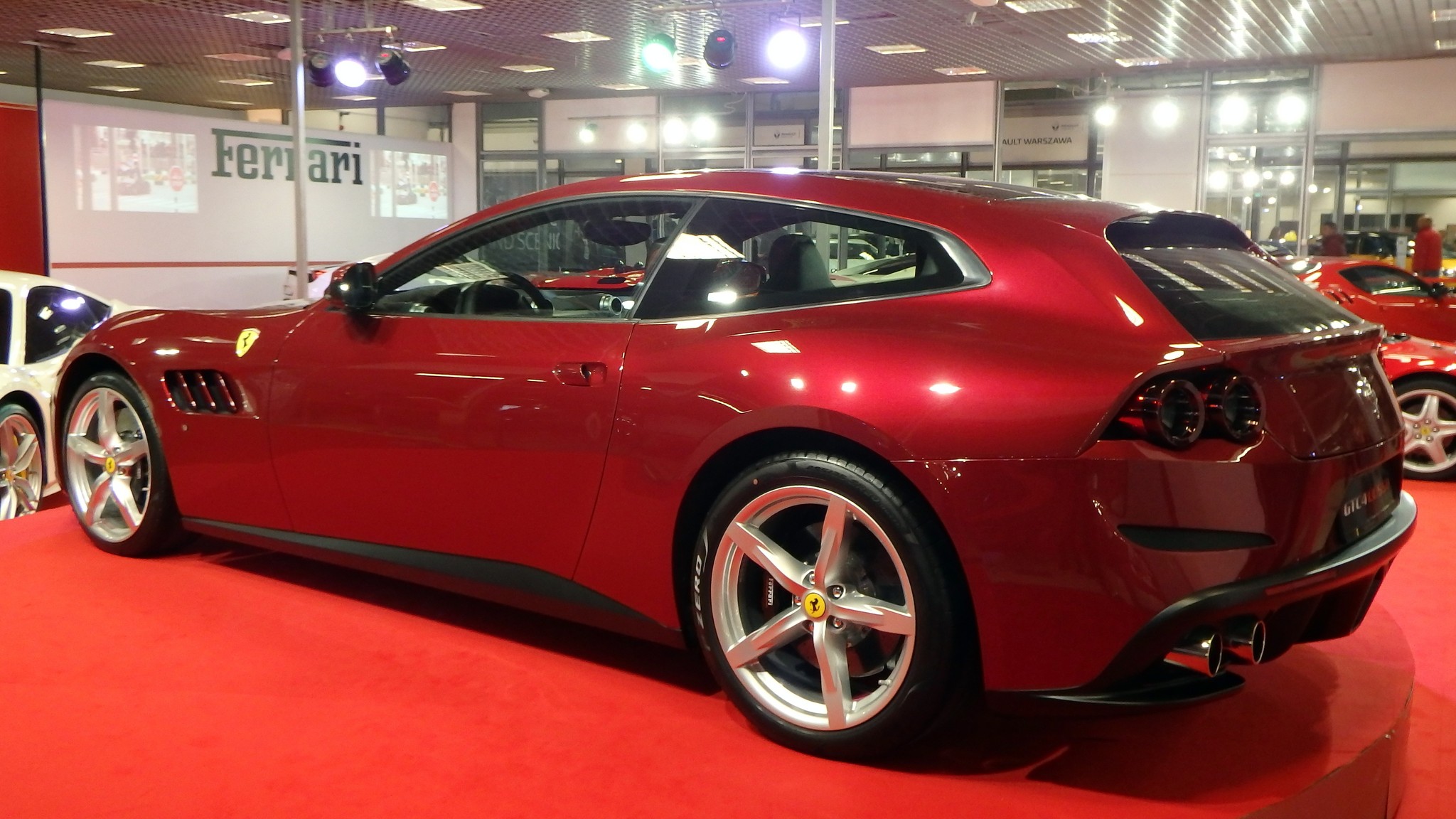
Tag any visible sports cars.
[2,167,1456,757]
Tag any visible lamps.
[702,10,738,70]
[765,6,809,70]
[639,15,678,73]
[308,22,413,90]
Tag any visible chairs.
[762,230,839,295]
[640,235,714,324]
